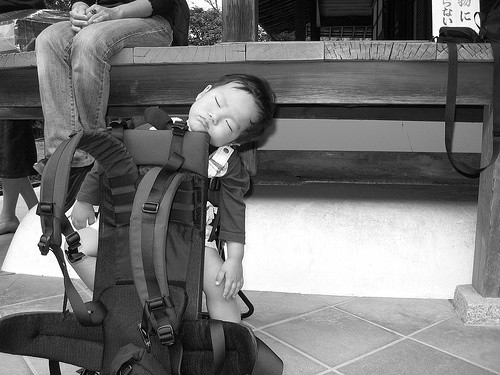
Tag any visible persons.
[67,74,276,324]
[0,0,46,234]
[34,0,190,172]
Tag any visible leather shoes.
[33,158,48,175]
[63,161,95,218]
